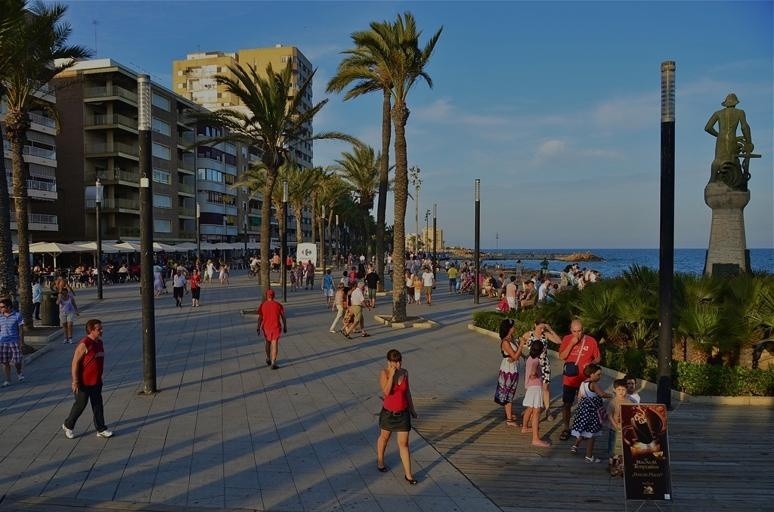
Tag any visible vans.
[530,271,563,289]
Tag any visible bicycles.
[247,265,260,279]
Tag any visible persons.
[29,250,141,287]
[321,251,436,339]
[497,315,639,475]
[152,258,230,308]
[372,347,421,484]
[55,286,80,345]
[0,299,24,388]
[706,92,754,181]
[31,278,43,320]
[248,251,280,276]
[443,251,600,313]
[255,289,286,371]
[286,254,315,290]
[61,318,113,438]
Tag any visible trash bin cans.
[40,290,59,326]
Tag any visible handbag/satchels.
[563,362,577,376]
[597,407,608,424]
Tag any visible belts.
[383,408,408,416]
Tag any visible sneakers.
[64,338,72,344]
[2,381,10,386]
[266,358,279,369]
[61,424,74,439]
[329,329,368,339]
[97,431,112,438]
[16,372,24,381]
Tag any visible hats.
[267,290,273,296]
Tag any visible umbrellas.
[12,240,279,270]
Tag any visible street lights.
[95,178,104,299]
[321,205,325,276]
[136,74,161,392]
[409,165,423,257]
[196,202,201,259]
[432,204,437,290]
[334,212,340,271]
[281,183,289,302]
[658,60,676,405]
[424,209,432,253]
[473,180,482,305]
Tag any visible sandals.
[584,456,600,463]
[570,446,577,454]
[559,430,570,440]
[377,464,387,472]
[405,474,417,484]
[504,411,554,447]
